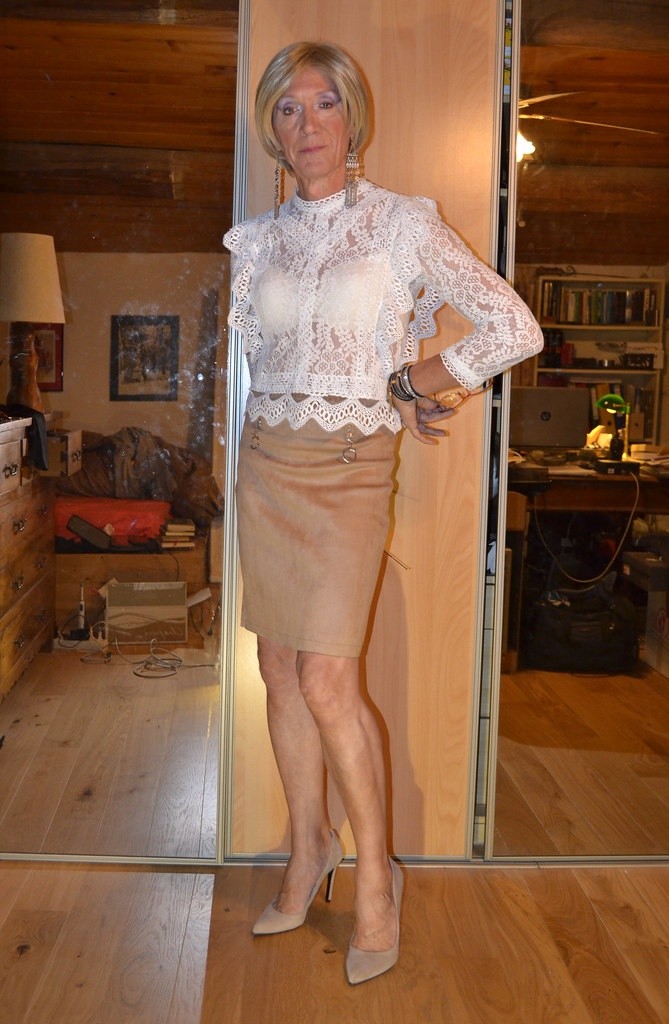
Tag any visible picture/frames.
[30,322,63,392]
[110,314,179,402]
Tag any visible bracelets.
[390,365,425,401]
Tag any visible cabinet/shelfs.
[528,275,669,446]
[0,410,84,706]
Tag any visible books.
[542,282,656,326]
[567,376,655,438]
[539,332,575,367]
[630,444,662,461]
[155,517,196,548]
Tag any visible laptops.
[509,386,590,447]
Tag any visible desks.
[500,475,669,674]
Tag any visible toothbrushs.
[78,584,85,629]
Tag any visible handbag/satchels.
[521,590,638,674]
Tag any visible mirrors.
[0,0,249,869]
[477,0,669,862]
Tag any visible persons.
[223,40,544,986]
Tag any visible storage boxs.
[97,579,212,644]
[628,413,644,439]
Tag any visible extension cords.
[53,636,105,651]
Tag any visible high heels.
[344,856,404,985]
[250,828,345,935]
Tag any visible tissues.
[66,514,115,551]
[581,426,613,459]
